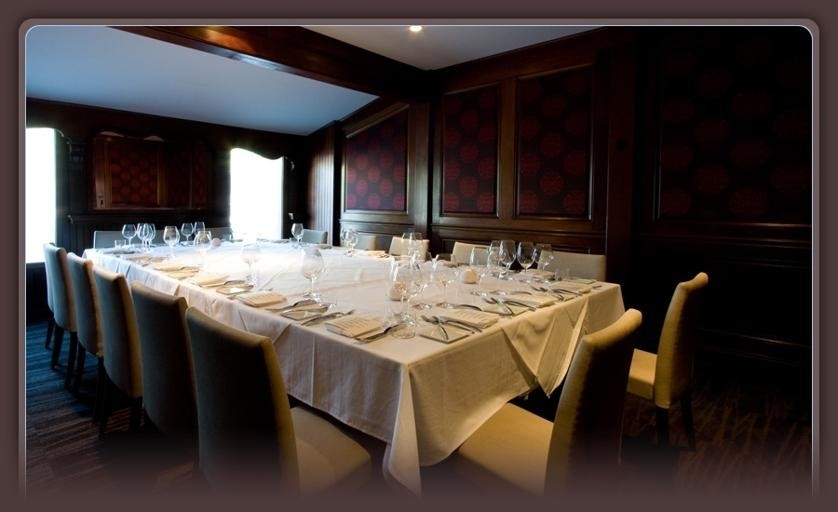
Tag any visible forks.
[310,310,354,321]
[532,285,570,293]
[482,297,532,309]
[421,315,473,334]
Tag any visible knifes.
[300,313,338,326]
[365,325,398,341]
[495,297,513,315]
[439,315,481,332]
[540,287,562,300]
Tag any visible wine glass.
[114,220,212,259]
[291,222,304,253]
[241,243,261,283]
[340,227,359,257]
[301,250,322,299]
[387,232,554,339]
